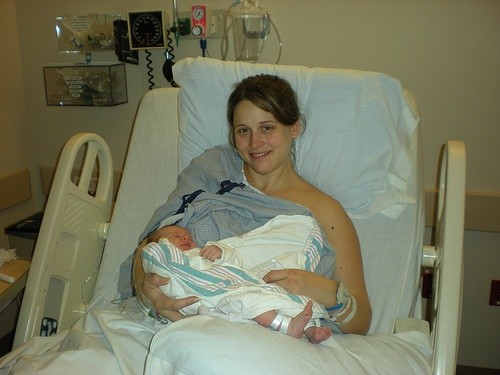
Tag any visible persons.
[145,225,332,344]
[132,74,373,336]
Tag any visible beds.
[0,56,467,375]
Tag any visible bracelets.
[327,280,358,327]
